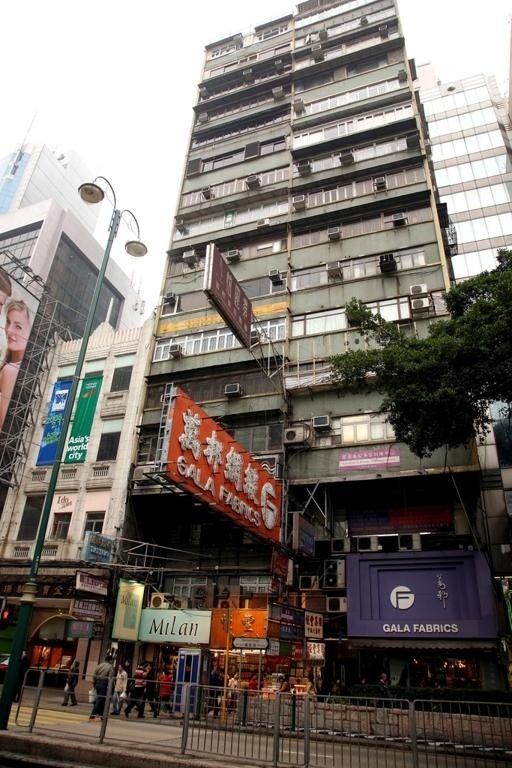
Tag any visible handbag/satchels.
[88,690,98,703]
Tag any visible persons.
[1,266,13,313]
[0,298,32,432]
[12,649,31,703]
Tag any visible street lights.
[4,176,147,731]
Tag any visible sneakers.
[61,703,174,722]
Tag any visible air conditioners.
[292,97,304,113]
[275,59,283,70]
[282,415,331,443]
[225,249,241,262]
[392,212,406,227]
[339,148,353,165]
[164,291,176,304]
[242,68,255,82]
[169,344,182,356]
[406,130,420,148]
[379,24,388,39]
[380,252,398,273]
[225,383,243,396]
[326,262,344,281]
[198,113,210,122]
[360,15,368,24]
[272,86,285,100]
[149,591,172,608]
[245,175,260,189]
[133,467,156,484]
[409,283,430,313]
[268,268,283,286]
[327,226,343,242]
[175,218,186,231]
[299,533,423,613]
[200,86,209,98]
[182,249,198,263]
[374,176,386,190]
[319,29,328,40]
[311,43,324,62]
[398,68,407,81]
[257,217,271,227]
[297,160,311,175]
[292,194,306,211]
[201,185,212,199]
